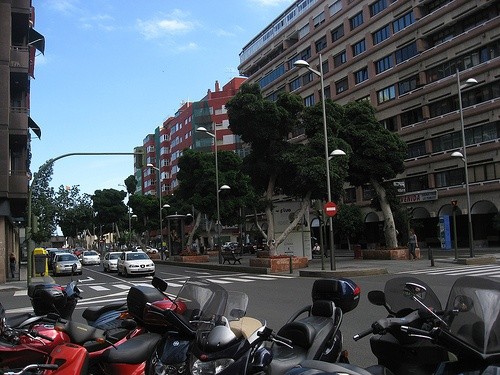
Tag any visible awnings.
[29,117,41,139]
[29,28,45,55]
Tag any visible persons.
[313,243,320,254]
[407,229,418,260]
[10,254,16,278]
[200,243,204,254]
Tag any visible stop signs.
[324,202,337,216]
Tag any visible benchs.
[312,246,327,258]
[221,251,242,265]
[164,251,176,261]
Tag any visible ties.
[315,246,317,250]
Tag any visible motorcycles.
[0,273,500,375]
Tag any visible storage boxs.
[28,283,65,318]
[312,276,360,314]
[127,285,174,328]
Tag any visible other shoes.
[414,256,416,259]
[12,276,14,278]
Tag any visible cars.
[103,251,123,272]
[136,246,159,254]
[223,242,256,250]
[118,251,156,276]
[46,247,101,275]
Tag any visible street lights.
[146,160,171,260]
[293,53,347,270]
[451,68,480,257]
[196,122,231,263]
